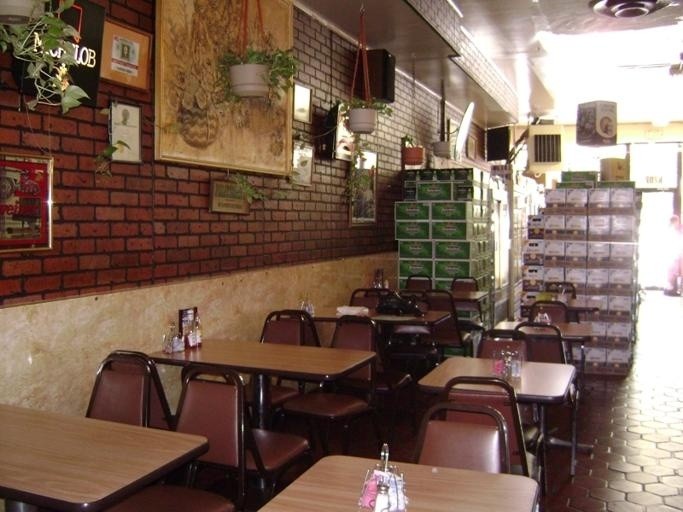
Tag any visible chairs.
[476,326,542,428]
[84,345,310,512]
[439,375,544,497]
[242,308,377,457]
[546,281,585,357]
[420,401,510,477]
[529,300,570,324]
[348,273,485,372]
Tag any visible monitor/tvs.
[329,102,355,161]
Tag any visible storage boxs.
[575,99,619,148]
[390,168,547,327]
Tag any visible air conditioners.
[526,122,565,176]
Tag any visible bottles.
[533,307,551,327]
[372,483,391,512]
[162,313,202,354]
[492,349,522,382]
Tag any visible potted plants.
[337,92,392,134]
[399,134,422,164]
[208,38,297,108]
[0,0,91,117]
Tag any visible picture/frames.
[292,80,313,126]
[288,139,313,187]
[447,118,464,166]
[203,179,251,216]
[107,100,143,164]
[0,150,55,254]
[466,133,478,161]
[99,17,153,94]
[152,0,294,178]
[348,146,377,227]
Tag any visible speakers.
[487,128,509,160]
[360,50,397,103]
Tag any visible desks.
[148,335,378,431]
[518,305,600,318]
[0,404,211,511]
[417,356,578,498]
[519,170,647,380]
[256,455,543,511]
[395,290,489,321]
[304,303,450,358]
[488,320,595,405]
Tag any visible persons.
[664,216,683,296]
